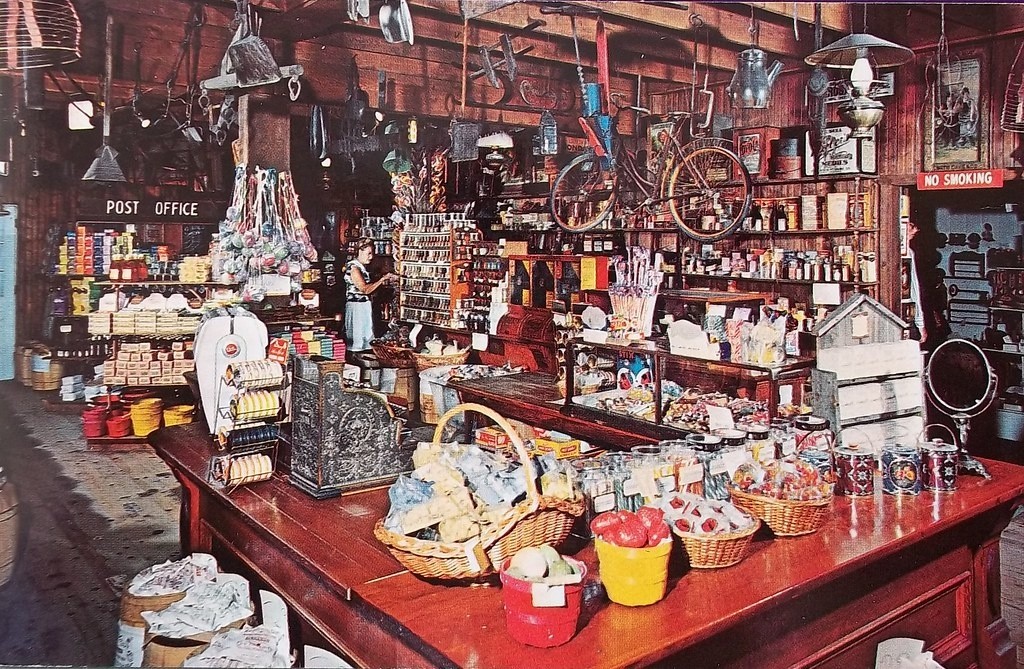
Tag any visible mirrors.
[925,338,1002,479]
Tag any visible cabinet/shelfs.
[45,217,239,412]
[83,273,338,456]
[393,173,884,450]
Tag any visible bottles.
[563,414,836,538]
[771,256,851,282]
[77,389,196,438]
[451,230,505,333]
[750,201,788,232]
[374,241,391,255]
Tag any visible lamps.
[132,91,151,128]
[361,110,387,138]
[477,132,514,161]
[804,33,916,138]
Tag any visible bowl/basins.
[378,0,414,47]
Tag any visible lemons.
[505,544,570,579]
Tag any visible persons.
[344,238,395,349]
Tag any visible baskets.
[726,471,832,536]
[670,504,761,569]
[375,402,584,578]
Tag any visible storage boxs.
[692,112,735,150]
[804,122,881,173]
[49,220,215,391]
[732,126,781,178]
[476,424,510,449]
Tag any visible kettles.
[727,47,785,109]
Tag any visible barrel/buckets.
[777,138,798,156]
[994,408,1024,441]
[881,442,921,496]
[499,555,588,649]
[594,535,675,607]
[833,442,876,495]
[794,446,833,482]
[772,156,801,178]
[920,438,960,490]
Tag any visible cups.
[702,215,724,231]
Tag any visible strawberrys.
[590,507,670,548]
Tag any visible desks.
[450,370,687,451]
[146,423,413,659]
[349,449,1024,669]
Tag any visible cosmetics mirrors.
[921,339,998,479]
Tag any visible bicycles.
[548,95,752,245]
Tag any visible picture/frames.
[923,45,990,171]
[647,116,683,181]
